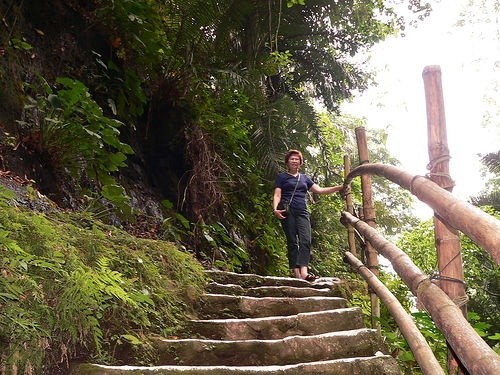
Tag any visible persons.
[272,148,351,282]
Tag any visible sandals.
[304,275,315,281]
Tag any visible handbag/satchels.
[277,201,291,217]
